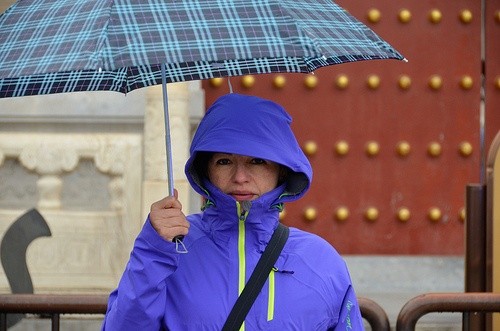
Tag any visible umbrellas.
[0,0,408,254]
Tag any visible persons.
[103,94,364,331]
